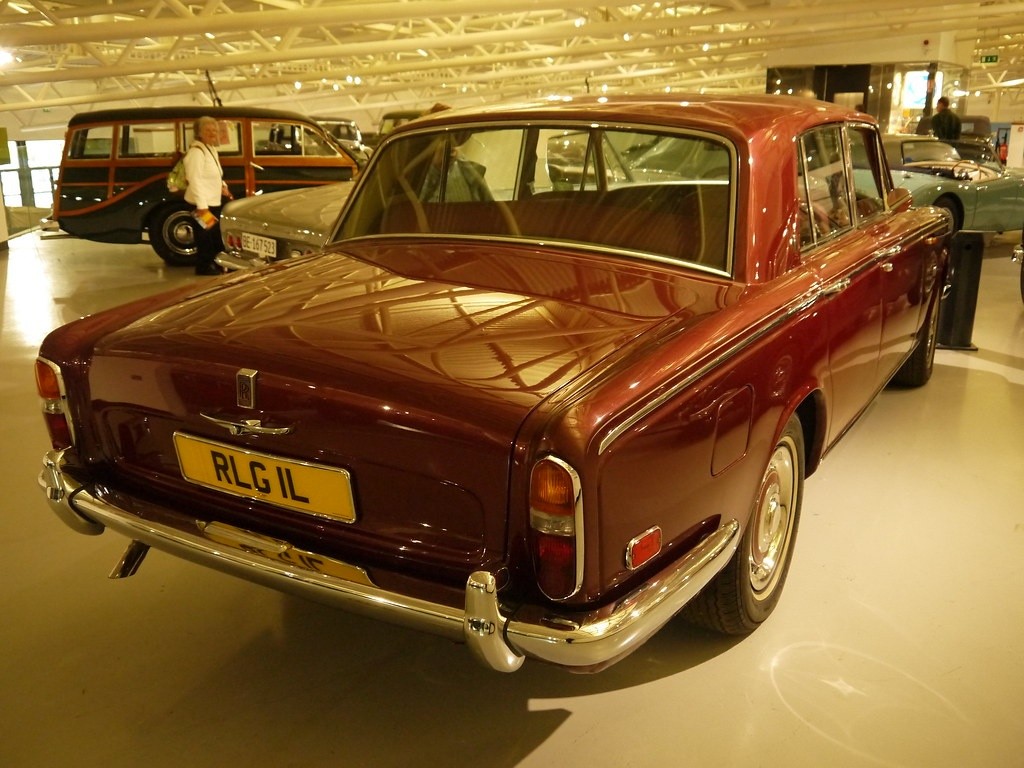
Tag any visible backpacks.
[166,155,188,194]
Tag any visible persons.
[916,108,933,135]
[933,97,961,139]
[183,117,227,276]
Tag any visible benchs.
[386,185,727,270]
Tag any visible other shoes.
[194,264,236,276]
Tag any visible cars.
[626,127,1022,232]
[34,87,957,677]
[40,103,363,265]
[309,116,375,160]
[212,112,699,275]
